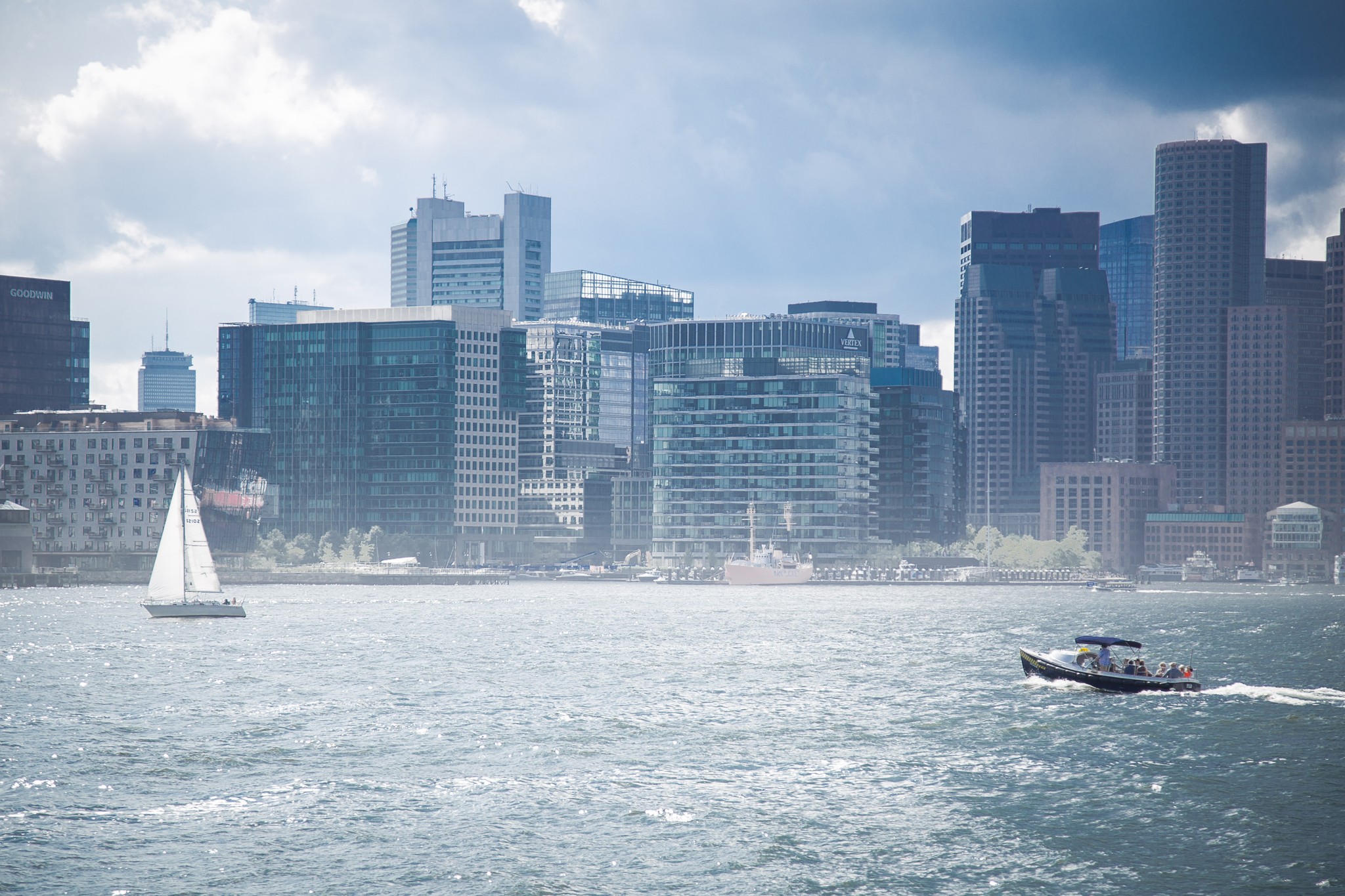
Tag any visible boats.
[626,568,675,585]
[1019,636,1202,693]
[1182,550,1220,582]
[515,571,554,581]
[555,568,591,581]
[1237,559,1264,582]
[1092,581,1137,592]
[1266,572,1329,585]
[724,496,814,586]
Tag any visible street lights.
[387,552,390,574]
[429,552,432,574]
[463,552,469,574]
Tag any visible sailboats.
[140,462,246,618]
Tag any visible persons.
[1136,660,1148,677]
[1162,662,1184,679]
[1184,666,1193,677]
[1124,660,1136,675]
[1134,659,1153,676]
[1154,662,1168,678]
[1178,664,1191,678]
[1099,645,1111,672]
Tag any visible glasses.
[1160,665,1163,667]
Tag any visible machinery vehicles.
[612,549,641,566]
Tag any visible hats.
[1179,664,1184,668]
[1169,662,1177,665]
[1134,658,1140,662]
